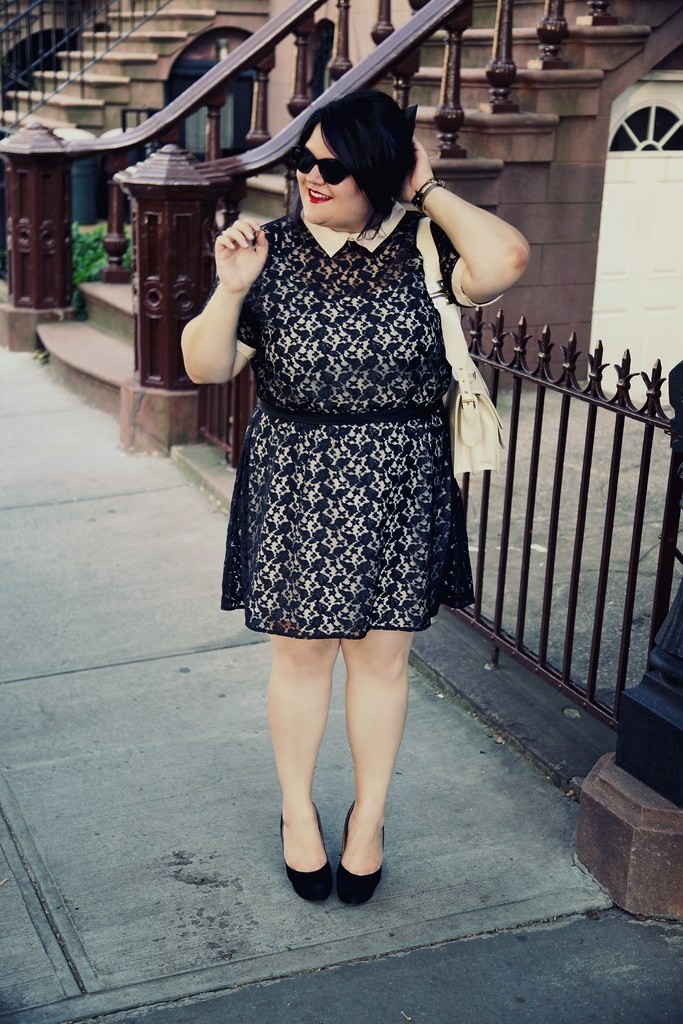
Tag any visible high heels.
[279,801,331,902]
[336,798,386,905]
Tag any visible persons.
[177,83,532,906]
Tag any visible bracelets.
[411,179,446,213]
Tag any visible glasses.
[291,144,351,185]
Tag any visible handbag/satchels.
[416,216,506,478]
